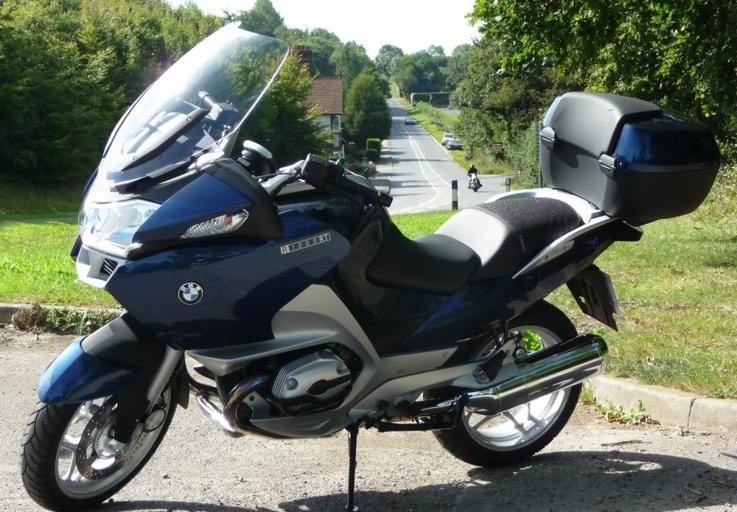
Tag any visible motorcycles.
[464,172,481,192]
[16,23,719,510]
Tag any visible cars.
[440,132,454,145]
[446,138,462,151]
[404,117,416,124]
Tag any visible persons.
[467,165,482,188]
